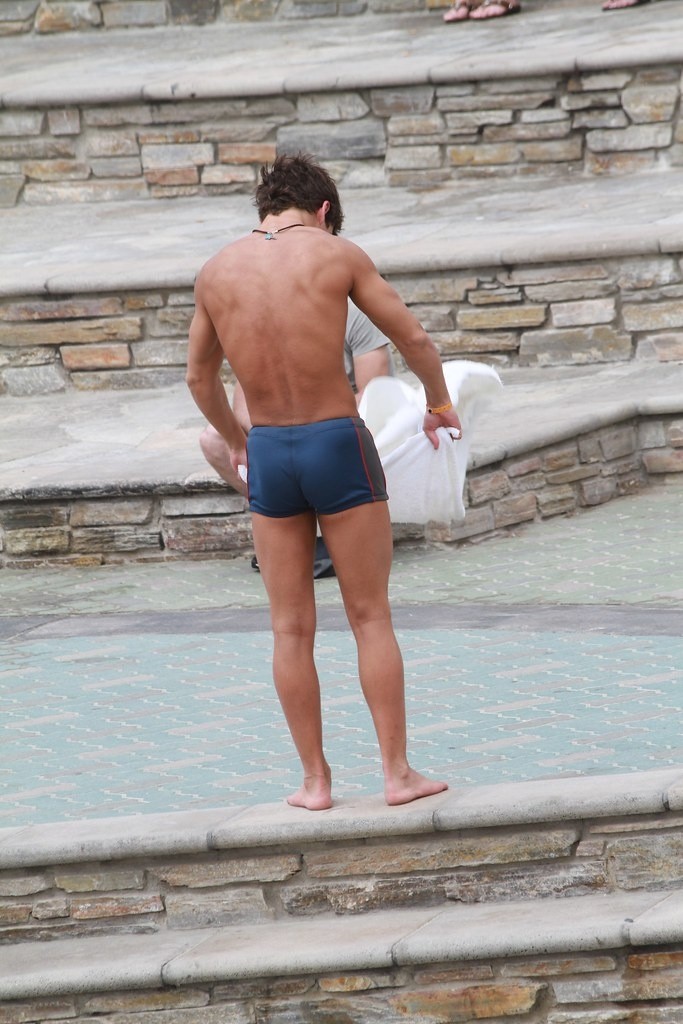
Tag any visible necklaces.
[251,222,304,241]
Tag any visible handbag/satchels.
[251,535,337,579]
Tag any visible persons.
[198,294,394,579]
[183,152,465,809]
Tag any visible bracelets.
[424,403,454,416]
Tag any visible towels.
[235,357,505,527]
